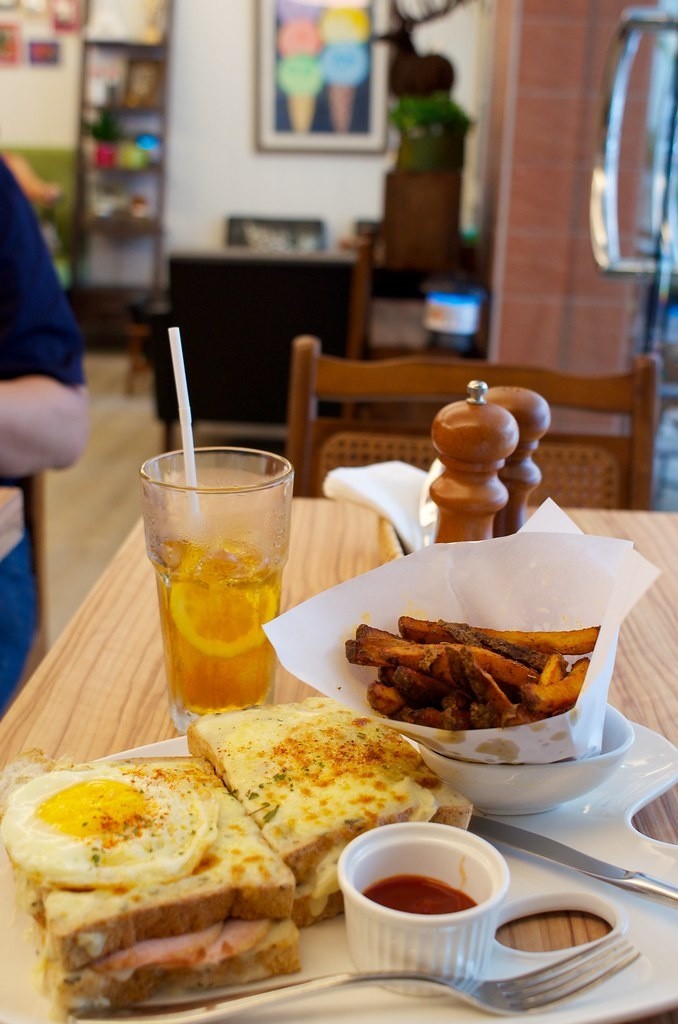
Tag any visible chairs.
[0,469,50,717]
[287,335,661,512]
[150,247,360,456]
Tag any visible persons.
[0,153,90,716]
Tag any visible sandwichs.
[185,699,474,928]
[30,757,303,1017]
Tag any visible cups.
[336,820,511,995]
[140,447,294,735]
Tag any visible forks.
[64,931,641,1022]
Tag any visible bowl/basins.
[418,702,634,816]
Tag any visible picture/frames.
[258,0,391,153]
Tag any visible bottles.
[483,385,551,542]
[430,380,520,545]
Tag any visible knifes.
[465,814,677,908]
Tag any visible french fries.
[345,614,599,730]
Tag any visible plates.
[0,720,678,1023]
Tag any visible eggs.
[1,745,221,890]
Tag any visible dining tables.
[0,499,678,1024]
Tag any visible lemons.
[168,549,277,658]
